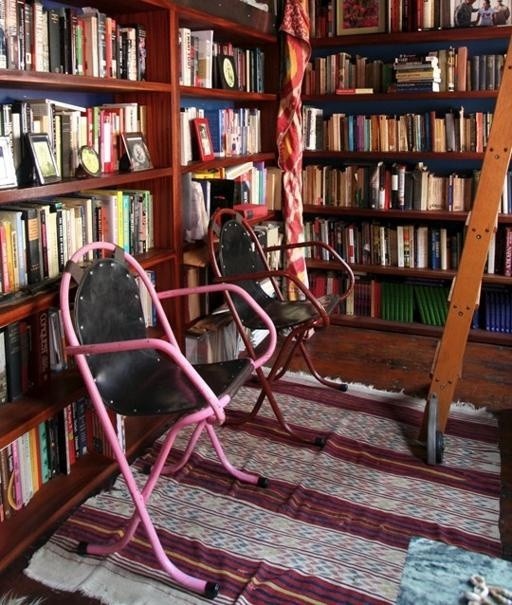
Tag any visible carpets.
[22,366,503,605]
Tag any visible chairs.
[207,208,355,446]
[60,241,277,599]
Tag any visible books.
[301,0,511,334]
[2,0,282,308]
[1,256,242,519]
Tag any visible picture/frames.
[440,0,512,29]
[120,132,154,173]
[75,145,102,179]
[27,132,62,186]
[216,54,238,90]
[336,0,386,36]
[192,118,218,161]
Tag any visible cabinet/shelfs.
[0,0,183,573]
[302,25,512,348]
[175,5,280,253]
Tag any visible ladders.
[416,33,511,465]
